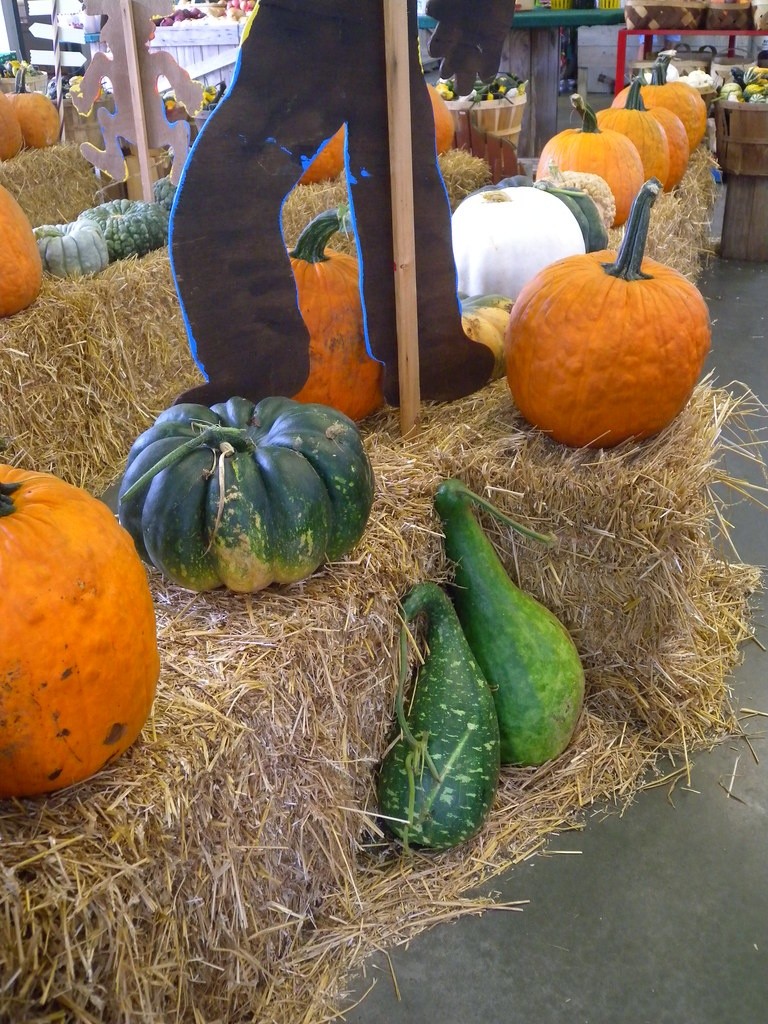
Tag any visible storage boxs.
[713,99,768,178]
[624,0,768,31]
[435,71,526,165]
[86,3,248,101]
[627,43,768,84]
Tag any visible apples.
[210,0,257,20]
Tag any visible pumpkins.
[0,49,768,855]
